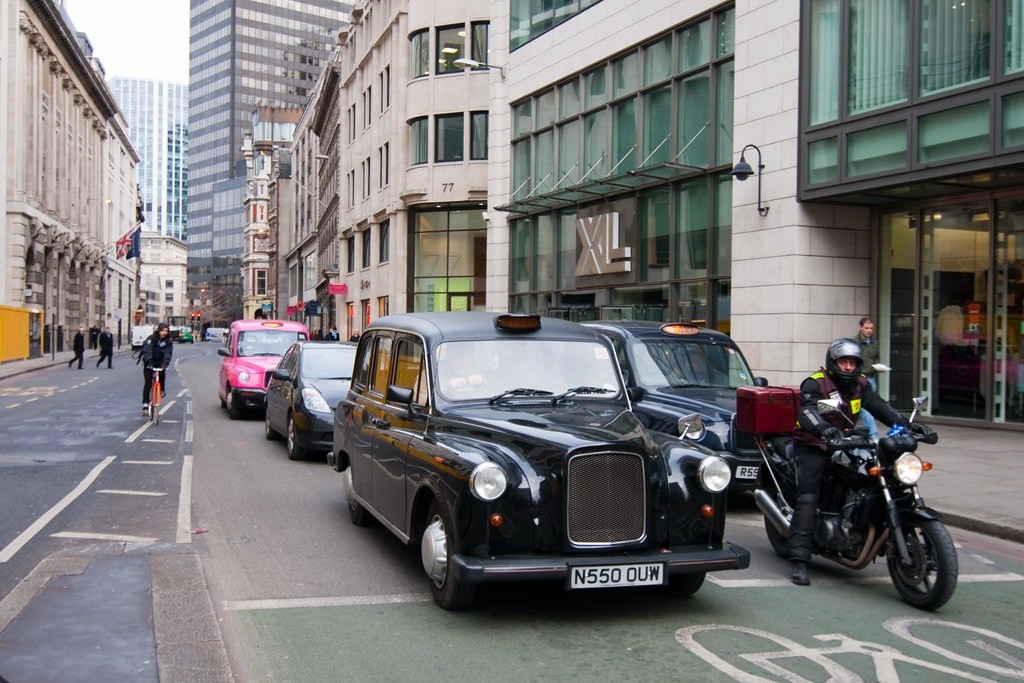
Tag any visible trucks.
[131,326,153,351]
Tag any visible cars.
[264,340,360,461]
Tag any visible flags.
[116,228,140,260]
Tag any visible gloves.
[822,427,844,441]
[909,423,938,445]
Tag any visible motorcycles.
[735,383,959,611]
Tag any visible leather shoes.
[791,563,810,585]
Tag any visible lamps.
[315,154,329,160]
[731,144,769,217]
[453,59,505,76]
[87,198,112,204]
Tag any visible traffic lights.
[197,311,201,317]
[191,313,195,316]
[200,287,205,293]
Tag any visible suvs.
[325,311,752,614]
[574,319,797,498]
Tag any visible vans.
[178,326,194,344]
[217,319,312,421]
[206,328,229,342]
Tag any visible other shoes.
[142,405,148,417]
[108,366,113,369]
[78,367,83,369]
[69,361,72,366]
[96,363,99,367]
[161,391,166,397]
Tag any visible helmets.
[825,339,865,389]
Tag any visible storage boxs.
[735,384,800,434]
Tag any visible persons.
[350,329,360,342]
[92,325,101,350]
[790,339,934,585]
[449,375,481,392]
[140,322,173,417]
[96,327,114,368]
[851,318,880,444]
[310,327,340,341]
[69,327,85,369]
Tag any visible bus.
[168,316,186,338]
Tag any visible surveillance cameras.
[482,212,491,222]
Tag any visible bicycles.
[146,366,170,427]
